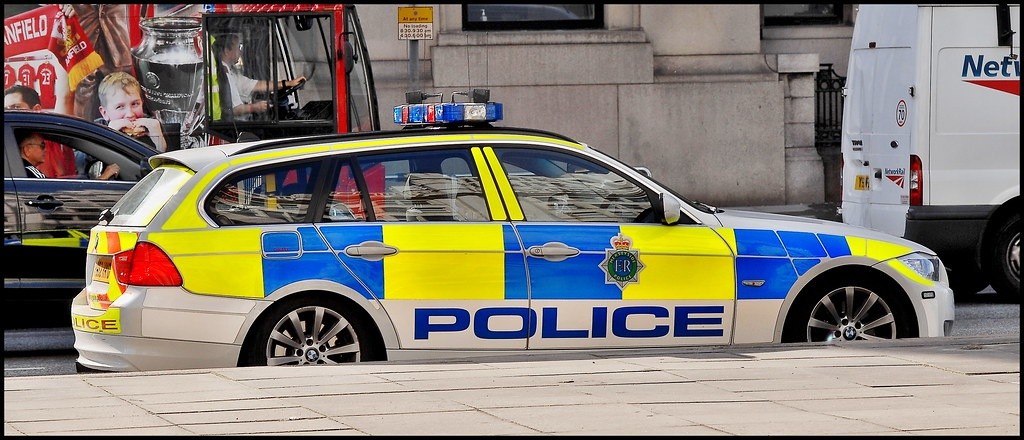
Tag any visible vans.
[839,2,1021,308]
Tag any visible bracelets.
[250,104,253,113]
[281,80,286,88]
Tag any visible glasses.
[28,142,46,150]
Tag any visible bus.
[3,3,380,160]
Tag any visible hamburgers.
[121,125,145,137]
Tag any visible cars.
[73,98,959,373]
[3,109,359,317]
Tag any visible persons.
[214,34,306,122]
[18,127,120,182]
[4,85,41,112]
[95,71,167,153]
